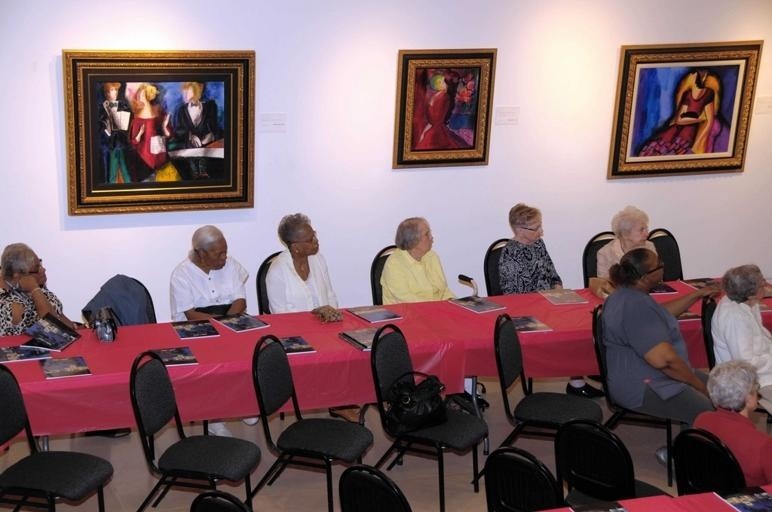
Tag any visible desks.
[0,277,768,452]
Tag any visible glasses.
[522,224,542,232]
[759,279,767,288]
[296,231,316,243]
[753,383,761,392]
[646,262,665,275]
[28,259,42,274]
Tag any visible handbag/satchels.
[386,370,448,432]
[589,277,617,299]
[310,304,344,324]
[90,307,118,342]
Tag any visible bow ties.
[191,102,198,106]
[109,102,118,108]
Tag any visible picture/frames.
[608,40,763,179]
[63,49,255,216]
[393,49,498,170]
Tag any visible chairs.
[371,323,486,512]
[555,421,673,502]
[484,240,516,295]
[484,446,563,512]
[470,314,611,477]
[127,351,260,511]
[701,297,771,423]
[584,230,615,287]
[186,490,249,512]
[257,251,286,313]
[670,429,750,498]
[0,364,114,512]
[371,246,401,304]
[245,335,371,511]
[338,467,413,512]
[593,302,692,433]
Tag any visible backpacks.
[90,306,122,343]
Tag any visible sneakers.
[85,427,131,438]
[208,419,232,437]
[241,416,259,425]
[655,446,674,469]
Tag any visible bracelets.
[23,287,45,295]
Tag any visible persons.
[175,82,225,181]
[681,277,724,291]
[169,225,260,437]
[674,307,703,322]
[691,360,772,489]
[496,203,606,400]
[417,68,473,150]
[379,216,489,409]
[264,213,369,422]
[710,264,771,419]
[601,248,722,465]
[130,83,173,182]
[638,63,732,159]
[0,244,132,439]
[596,205,664,283]
[92,80,126,187]
[638,274,677,295]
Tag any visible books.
[38,355,91,379]
[506,315,552,334]
[212,313,271,333]
[336,326,400,351]
[345,303,401,323]
[536,289,586,305]
[170,319,220,340]
[713,481,770,511]
[150,344,199,367]
[445,294,509,312]
[0,343,55,364]
[19,313,82,351]
[258,335,314,354]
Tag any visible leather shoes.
[329,406,366,423]
[566,382,605,398]
[460,389,490,407]
